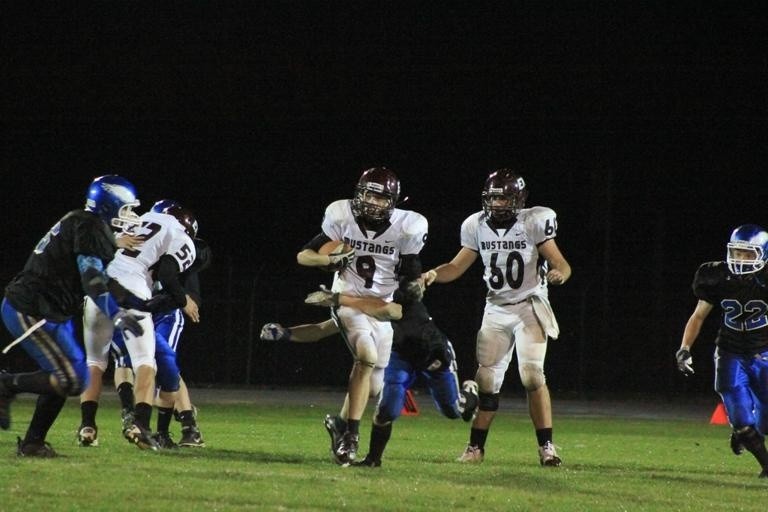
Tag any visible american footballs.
[318,241,352,273]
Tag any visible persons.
[259,258,477,468]
[296,164,426,468]
[674,224,767,480]
[423,169,573,465]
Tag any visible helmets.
[725,226,768,275]
[151,199,199,240]
[85,173,143,237]
[355,167,399,220]
[480,169,527,222]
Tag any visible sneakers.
[456,445,484,463]
[463,380,479,421]
[324,414,381,468]
[17,436,67,459]
[731,429,745,456]
[0,370,18,429]
[77,424,97,447]
[539,440,562,467]
[121,402,205,451]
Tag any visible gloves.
[676,345,695,376]
[328,244,355,270]
[407,269,426,302]
[113,310,146,340]
[260,322,291,342]
[304,284,339,307]
